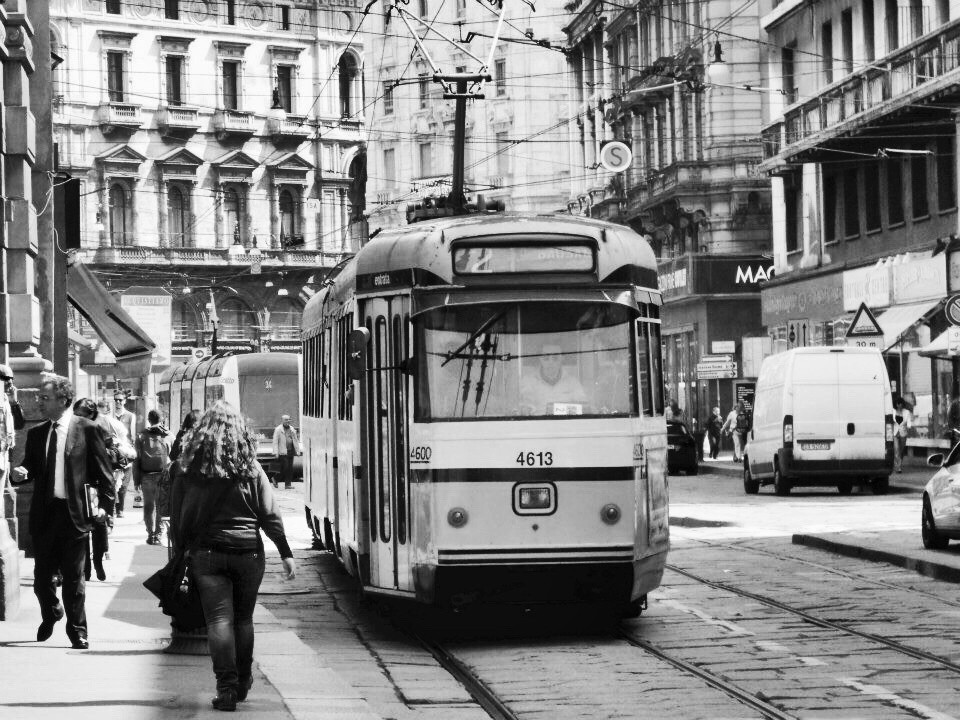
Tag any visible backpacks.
[139,432,169,472]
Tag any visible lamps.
[705,31,732,88]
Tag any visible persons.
[516,344,586,412]
[9,377,300,712]
[892,397,912,473]
[706,407,724,459]
[719,404,744,462]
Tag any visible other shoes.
[271,477,279,488]
[117,510,123,517]
[85,573,90,581]
[147,533,162,544]
[237,675,254,701]
[57,576,63,586]
[286,485,294,489]
[72,638,89,649]
[37,607,63,641]
[211,691,236,711]
[94,560,106,580]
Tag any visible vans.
[740,344,896,497]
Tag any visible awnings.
[874,298,943,348]
[64,262,156,381]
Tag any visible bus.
[156,284,304,481]
[294,8,677,638]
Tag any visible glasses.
[38,394,55,403]
[114,398,125,402]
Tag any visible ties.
[46,422,58,501]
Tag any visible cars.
[920,429,960,554]
[666,417,700,477]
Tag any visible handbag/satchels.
[159,460,177,516]
[162,565,196,618]
